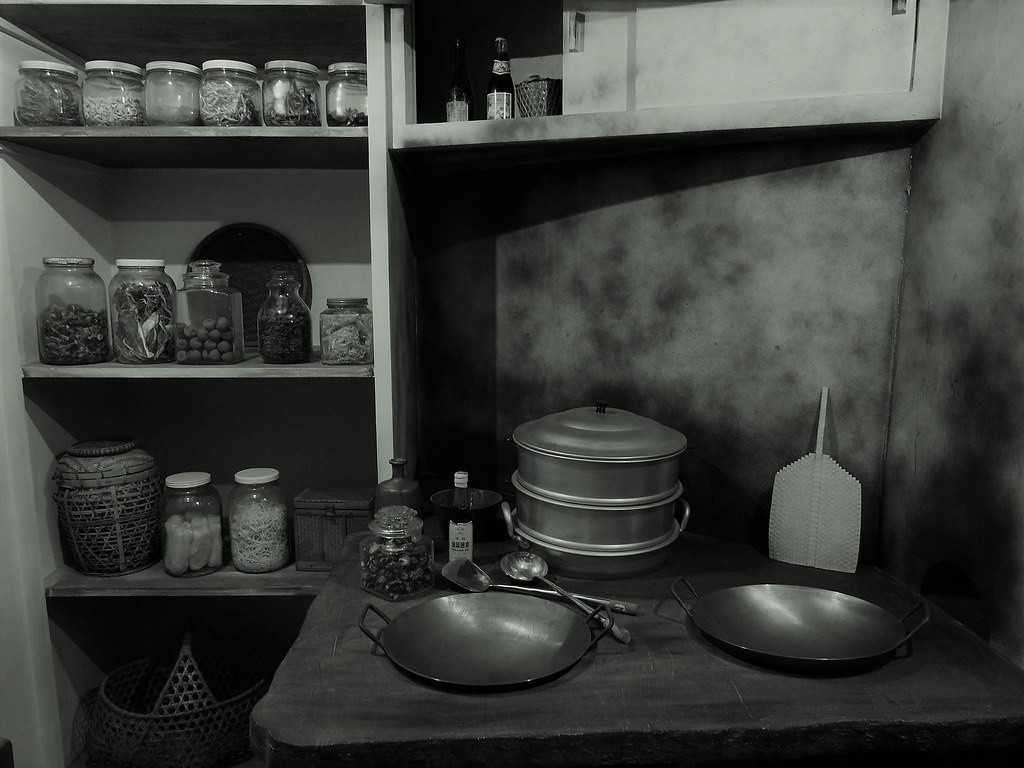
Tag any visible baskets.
[92,642,271,768]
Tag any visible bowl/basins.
[431,489,503,540]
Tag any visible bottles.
[162,470,223,578]
[229,467,292,573]
[448,470,473,566]
[485,36,514,121]
[444,35,474,120]
[360,458,439,603]
[11,59,367,126]
[34,256,371,364]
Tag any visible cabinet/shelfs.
[0,0,412,768]
[390,0,951,149]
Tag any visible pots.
[501,399,693,580]
[358,592,618,687]
[669,578,928,662]
